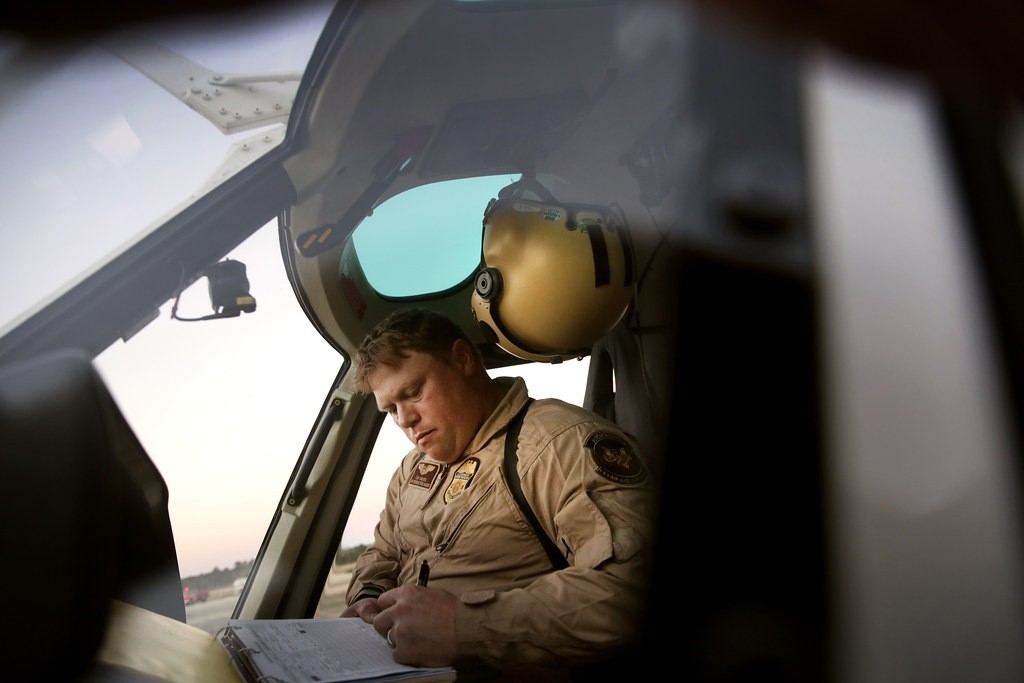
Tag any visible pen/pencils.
[417,560,430,587]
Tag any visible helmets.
[471,197,636,365]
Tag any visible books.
[98,600,456,683]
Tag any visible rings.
[388,629,395,646]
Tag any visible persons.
[339,310,658,683]
